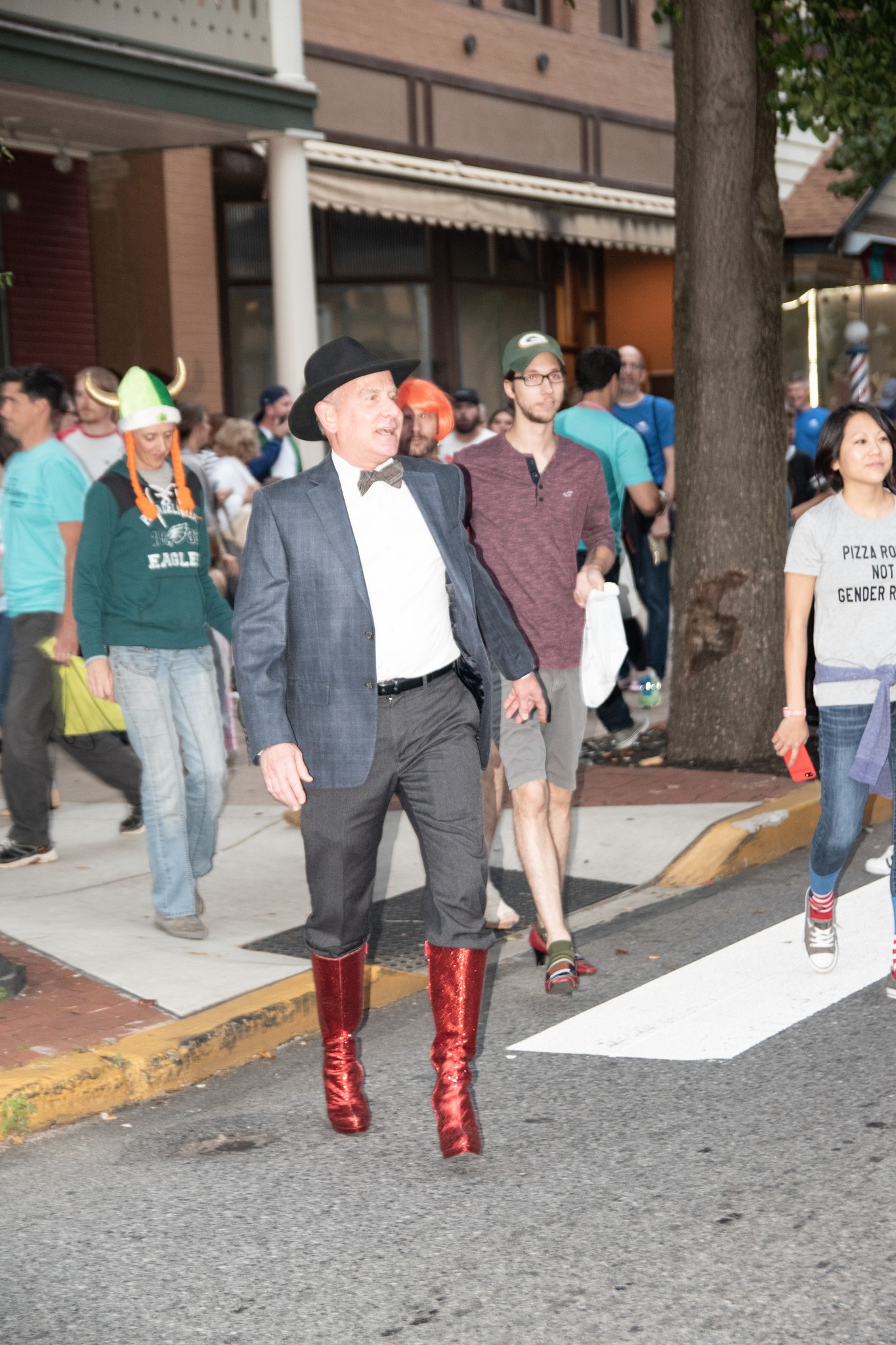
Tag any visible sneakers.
[883,971,896,1001]
[803,887,839,975]
[0,835,57,869]
[118,802,146,836]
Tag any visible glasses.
[513,370,565,387]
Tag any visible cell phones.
[774,730,817,782]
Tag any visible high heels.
[544,957,579,999]
[528,926,598,976]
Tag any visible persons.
[0,954,26,1002]
[231,338,547,1163]
[397,376,515,466]
[771,368,896,1000]
[551,344,674,751]
[0,363,146,867]
[455,331,617,1000]
[72,355,234,940]
[178,383,304,600]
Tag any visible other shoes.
[638,668,662,709]
[154,911,208,941]
[612,716,649,749]
[480,882,520,930]
[193,887,206,916]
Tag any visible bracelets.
[782,706,806,717]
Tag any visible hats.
[253,386,289,424]
[452,387,479,404]
[287,336,421,441]
[501,330,565,380]
[84,357,194,519]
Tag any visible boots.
[422,940,487,1159]
[309,944,371,1134]
